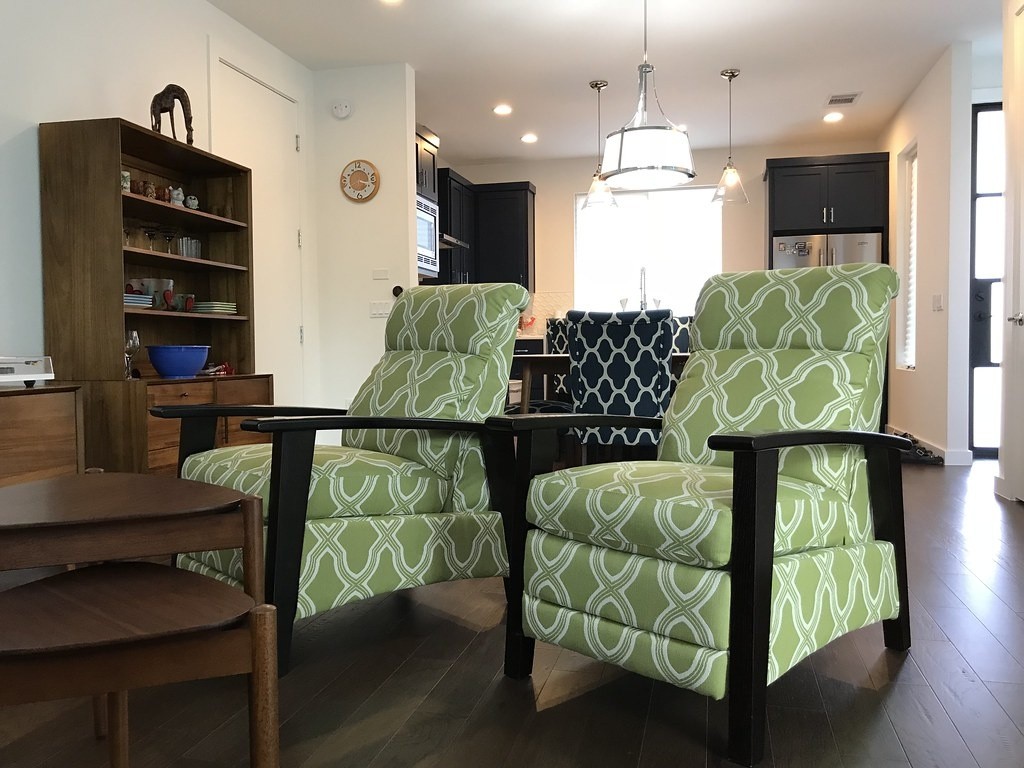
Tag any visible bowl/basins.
[144,345,212,379]
[173,293,195,312]
[126,278,174,310]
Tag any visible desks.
[0,466,283,767]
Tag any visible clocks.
[340,159,381,203]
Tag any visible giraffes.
[150,84,194,147]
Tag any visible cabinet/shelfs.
[36,115,275,479]
[766,151,890,235]
[0,384,84,488]
[436,175,535,296]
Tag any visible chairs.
[147,282,528,680]
[502,259,914,768]
[544,309,695,469]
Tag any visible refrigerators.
[772,233,882,270]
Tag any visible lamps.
[711,67,751,207]
[581,80,617,209]
[598,0,702,192]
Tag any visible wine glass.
[157,224,178,254]
[123,220,134,247]
[125,331,140,380]
[140,226,160,251]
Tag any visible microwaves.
[417,195,439,272]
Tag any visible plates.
[192,301,238,315]
[123,294,153,308]
